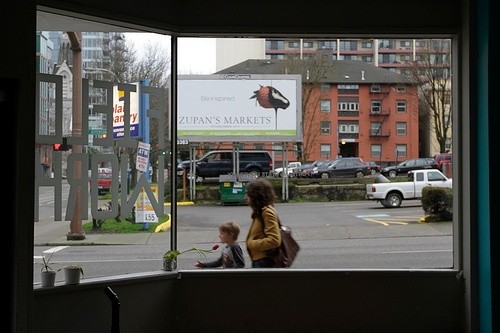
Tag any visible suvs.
[89,172,121,195]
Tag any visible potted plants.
[40,252,55,287]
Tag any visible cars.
[312,157,371,179]
[380,158,439,178]
[365,161,381,175]
[434,153,452,172]
[294,160,333,179]
[271,162,302,178]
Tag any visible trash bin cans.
[219,173,254,203]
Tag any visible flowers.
[167,245,220,260]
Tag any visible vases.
[163,257,176,271]
[64,267,80,285]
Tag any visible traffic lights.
[52,137,73,151]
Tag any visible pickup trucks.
[365,169,452,208]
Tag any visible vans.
[177,150,272,181]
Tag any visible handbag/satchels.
[267,226,300,267]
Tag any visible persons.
[246,178,282,269]
[195,221,245,269]
